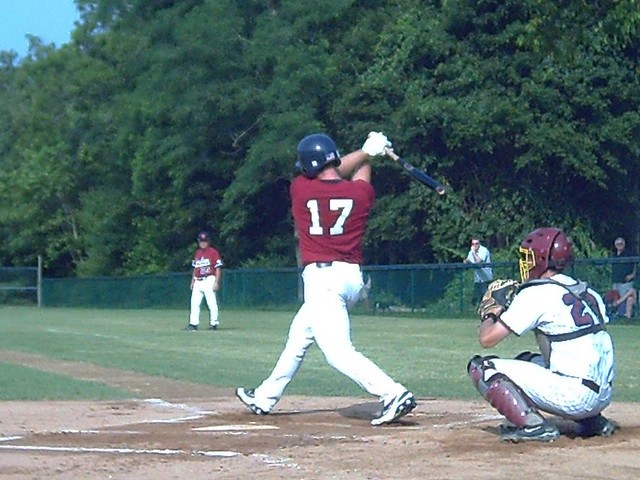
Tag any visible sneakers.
[574,417,620,437]
[207,325,218,330]
[371,387,416,425]
[236,387,270,415]
[184,324,198,331]
[499,424,559,441]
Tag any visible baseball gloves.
[477,279,522,318]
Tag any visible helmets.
[295,134,342,178]
[197,232,210,242]
[519,228,572,280]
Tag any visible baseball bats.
[367,131,446,195]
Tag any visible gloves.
[362,131,392,157]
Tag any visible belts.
[582,379,613,395]
[315,262,332,267]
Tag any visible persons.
[463,233,495,305]
[182,231,223,331]
[610,285,639,320]
[466,227,620,442]
[608,237,638,316]
[235,130,416,426]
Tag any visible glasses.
[472,242,479,245]
[616,243,624,245]
